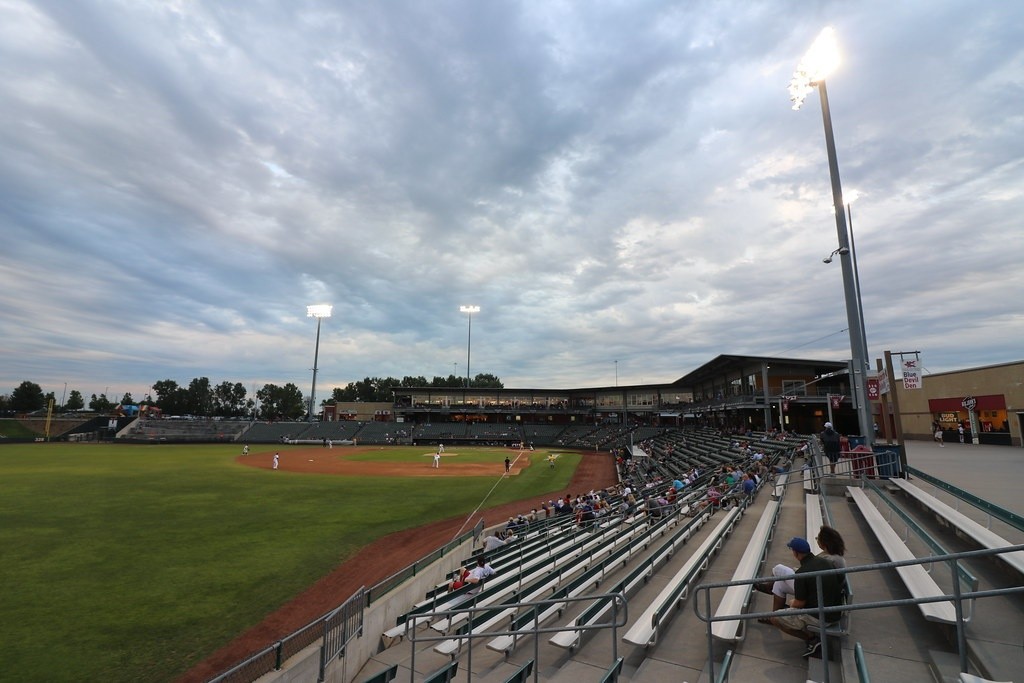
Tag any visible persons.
[432,453,440,469]
[934,420,945,447]
[503,529,518,543]
[323,437,333,448]
[439,431,452,439]
[505,457,510,472]
[873,421,880,439]
[447,555,495,593]
[272,452,280,469]
[957,423,967,443]
[506,508,538,532]
[770,537,842,658]
[820,422,841,477]
[386,420,425,446]
[243,444,250,455]
[438,443,444,454]
[488,423,536,451]
[542,419,812,533]
[482,531,508,554]
[279,433,290,444]
[753,524,847,625]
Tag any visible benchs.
[380,424,1024,683]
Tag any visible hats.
[682,473,688,477]
[714,468,721,472]
[532,508,537,512]
[959,424,962,426]
[824,422,832,428]
[787,538,810,553]
[510,517,513,521]
[517,515,521,519]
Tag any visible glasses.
[789,548,794,551]
[815,537,820,540]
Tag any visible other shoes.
[758,616,774,626]
[753,581,775,595]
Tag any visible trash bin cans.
[847,434,866,450]
[871,442,901,480]
[839,436,849,458]
[850,445,875,480]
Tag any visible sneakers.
[802,637,821,657]
